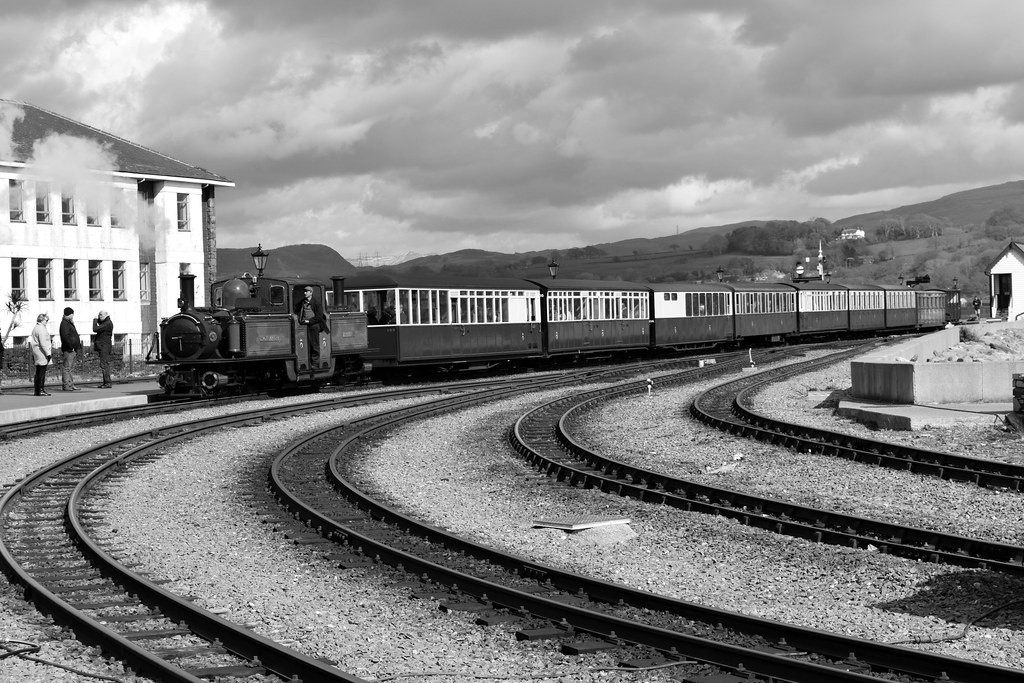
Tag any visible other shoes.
[73,386,81,390]
[63,387,74,391]
[98,382,113,388]
[311,365,320,370]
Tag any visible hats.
[64,308,74,315]
[303,286,313,292]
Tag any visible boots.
[33,375,51,396]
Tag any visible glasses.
[44,319,49,323]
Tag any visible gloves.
[46,355,52,362]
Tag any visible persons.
[93,309,114,388]
[59,307,81,391]
[296,286,330,370]
[973,295,981,320]
[31,314,54,396]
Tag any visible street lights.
[716,265,725,283]
[547,257,560,279]
[952,276,959,289]
[251,243,270,277]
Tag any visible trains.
[142,273,961,401]
